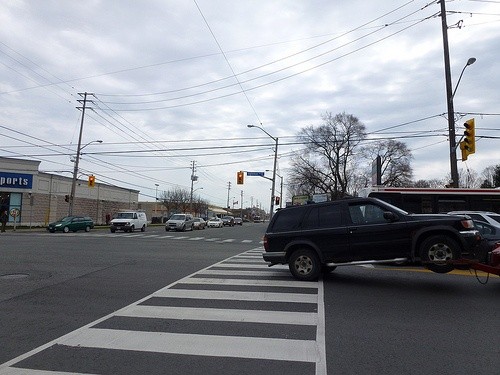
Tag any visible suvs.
[165,214,194,232]
[109,212,148,233]
[263,197,482,282]
[222,216,235,226]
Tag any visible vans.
[254,216,260,223]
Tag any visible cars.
[446,211,500,246]
[234,217,243,224]
[192,217,206,230]
[48,215,95,233]
[207,217,223,227]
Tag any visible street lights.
[190,188,204,214]
[265,170,283,208]
[445,58,477,188]
[247,124,278,224]
[68,140,103,216]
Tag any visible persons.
[0,211,8,232]
[105,212,110,225]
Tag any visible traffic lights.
[276,196,279,205]
[463,118,475,154]
[88,175,95,188]
[237,172,243,184]
[64,194,70,203]
[460,140,467,162]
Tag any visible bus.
[357,188,500,215]
